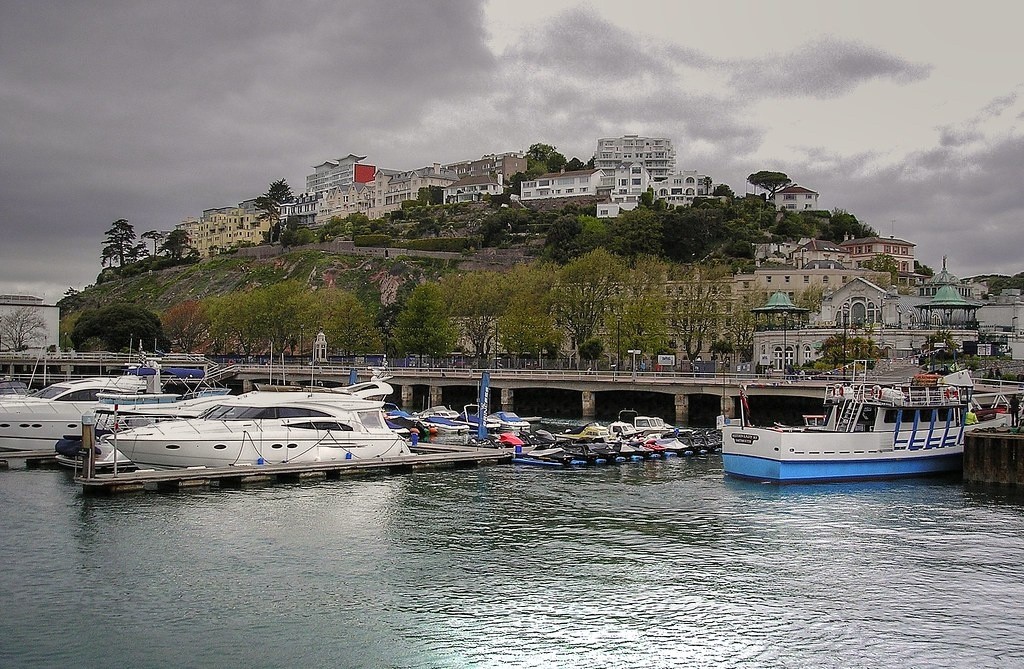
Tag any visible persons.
[635,358,663,376]
[1010,394,1019,427]
[756,362,773,377]
[787,365,803,380]
[965,409,979,425]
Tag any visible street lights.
[781,311,789,378]
[299,323,304,369]
[615,313,622,376]
[64,331,68,352]
[841,306,851,384]
[224,331,228,356]
[495,319,499,372]
[130,333,133,354]
[384,321,390,361]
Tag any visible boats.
[715,356,1024,487]
[0,337,724,474]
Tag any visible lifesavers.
[833,384,843,398]
[870,385,882,401]
[945,386,959,401]
